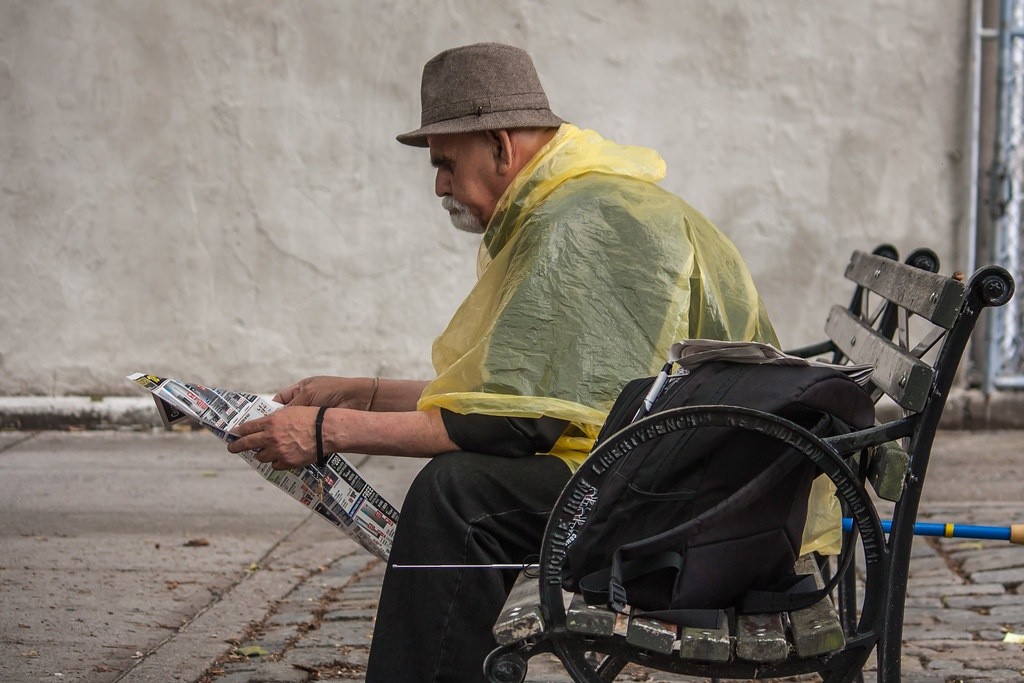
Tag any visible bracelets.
[315,406,330,465]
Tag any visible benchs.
[482,242,1014,683]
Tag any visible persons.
[225,42,784,683]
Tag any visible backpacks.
[520,360,876,630]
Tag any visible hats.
[395,43,571,148]
